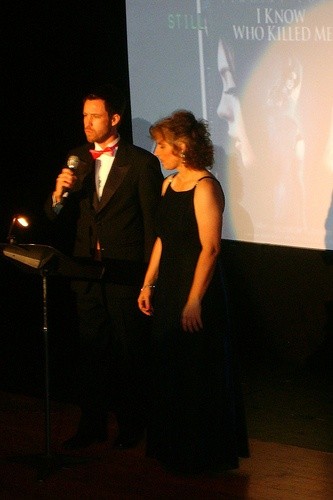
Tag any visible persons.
[45,85,164,449]
[136,113,251,479]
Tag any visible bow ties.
[88,142,119,160]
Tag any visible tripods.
[0,242,92,484]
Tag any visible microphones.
[61,156,80,204]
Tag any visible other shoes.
[62,433,108,451]
[111,433,143,448]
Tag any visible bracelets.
[140,285,153,293]
